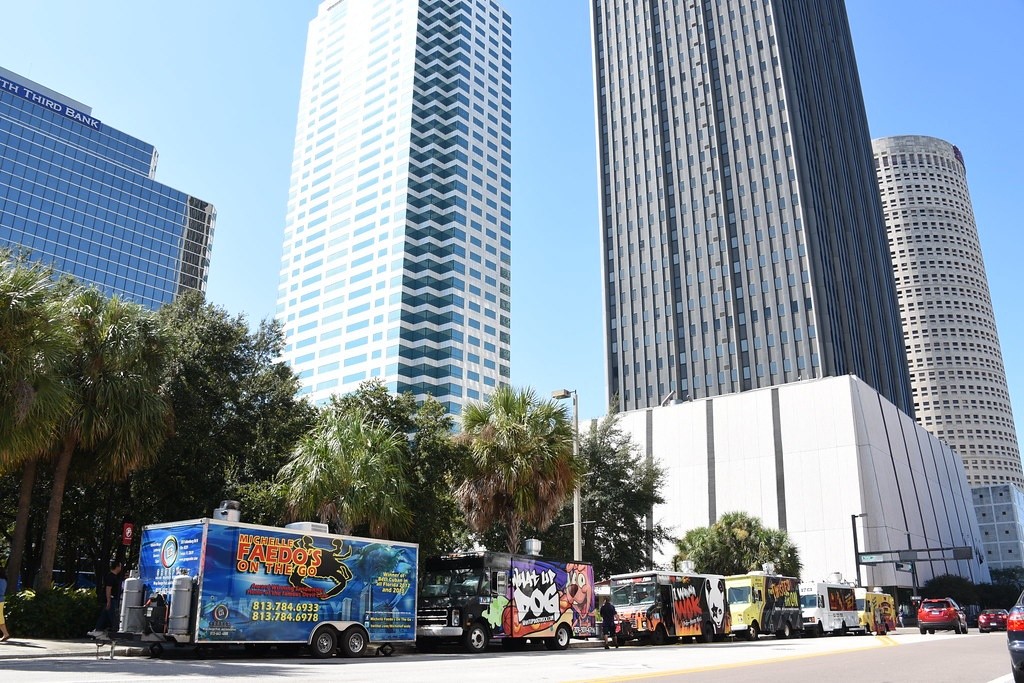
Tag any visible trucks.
[415,538,596,653]
[799,571,860,638]
[724,561,805,641]
[609,560,732,646]
[848,586,897,635]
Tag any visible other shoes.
[605,644,610,649]
[616,644,619,648]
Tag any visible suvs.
[918,598,968,634]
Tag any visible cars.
[978,609,1009,633]
[1007,589,1024,683]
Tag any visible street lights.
[551,389,582,561]
[851,513,868,588]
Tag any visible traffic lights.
[913,601,916,606]
[895,562,903,569]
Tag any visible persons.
[96,560,121,630]
[600,596,620,649]
[0,567,10,642]
